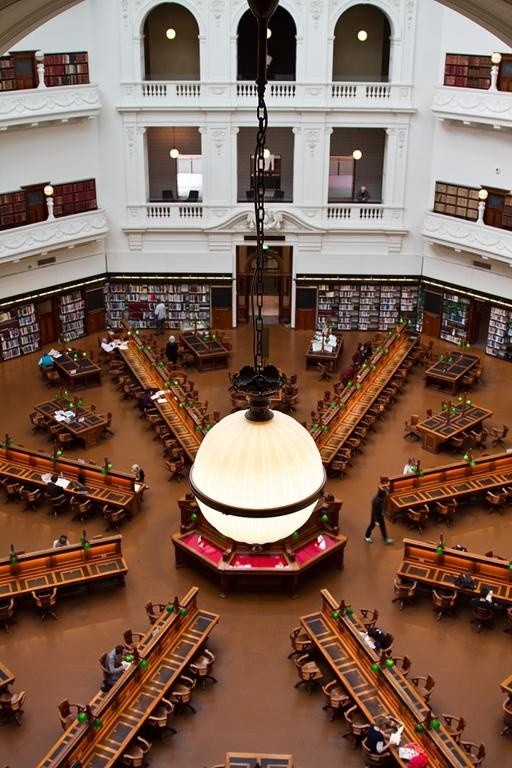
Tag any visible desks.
[375,448,511,537]
[1,531,131,623]
[173,325,232,374]
[402,392,511,456]
[37,344,106,389]
[422,339,487,397]
[98,326,223,483]
[303,328,345,375]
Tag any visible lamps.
[33,51,48,88]
[43,185,58,221]
[188,7,330,545]
[486,50,502,91]
[351,128,365,161]
[167,125,181,162]
[475,188,489,225]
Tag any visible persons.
[366,490,391,546]
[140,386,151,409]
[368,627,388,648]
[41,358,52,366]
[371,718,391,750]
[405,458,419,475]
[131,466,144,482]
[479,590,499,619]
[51,534,71,545]
[110,645,129,675]
[47,476,65,501]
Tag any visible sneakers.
[365,536,372,541]
[385,538,393,543]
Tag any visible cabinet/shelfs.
[438,287,481,346]
[0,188,30,234]
[313,278,425,330]
[1,299,43,362]
[42,50,91,87]
[101,276,214,331]
[443,51,494,89]
[485,303,511,363]
[431,180,480,222]
[0,56,18,92]
[52,178,99,218]
[501,192,512,232]
[54,286,89,343]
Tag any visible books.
[5,282,497,354]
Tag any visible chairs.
[0,630,220,766]
[300,328,434,481]
[286,653,512,768]
[1,432,151,532]
[391,537,511,637]
[223,361,302,413]
[27,391,116,452]
